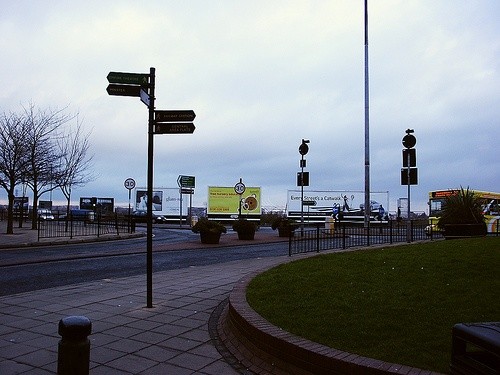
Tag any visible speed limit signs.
[125,178,135,189]
[235,183,245,194]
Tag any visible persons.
[424,224,431,239]
[137,191,160,210]
[374,204,386,221]
[331,203,339,221]
[341,193,349,212]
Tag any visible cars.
[59,213,68,219]
[131,211,167,225]
[359,199,383,211]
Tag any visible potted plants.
[233,217,258,239]
[191,213,227,246]
[270,216,298,238]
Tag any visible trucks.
[68,210,94,224]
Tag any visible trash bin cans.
[325,216,335,237]
[191,216,198,227]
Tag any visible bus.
[424,189,500,238]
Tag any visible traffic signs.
[179,176,195,187]
[303,201,317,206]
[106,72,196,136]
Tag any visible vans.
[28,207,55,221]
